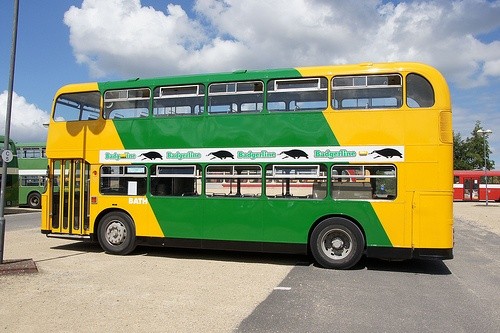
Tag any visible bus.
[40,62,454,271]
[13,141,91,209]
[452,171,500,204]
[0,136,19,207]
[196,168,370,197]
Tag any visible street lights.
[476,129,493,205]
